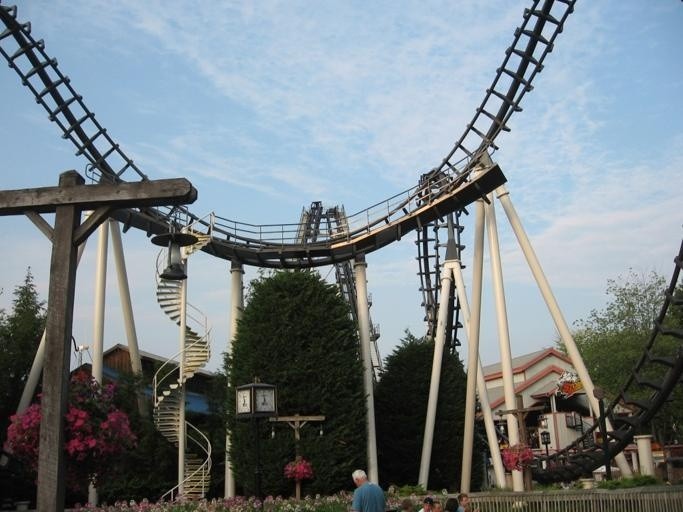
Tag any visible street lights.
[267,413,326,512]
[75,344,89,366]
[235,374,281,512]
[539,430,552,456]
[492,396,550,491]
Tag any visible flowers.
[500,443,533,471]
[6,368,137,491]
[283,455,313,480]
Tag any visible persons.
[457,493,480,512]
[430,500,443,512]
[399,499,414,512]
[443,497,458,512]
[418,497,433,512]
[350,468,384,512]
